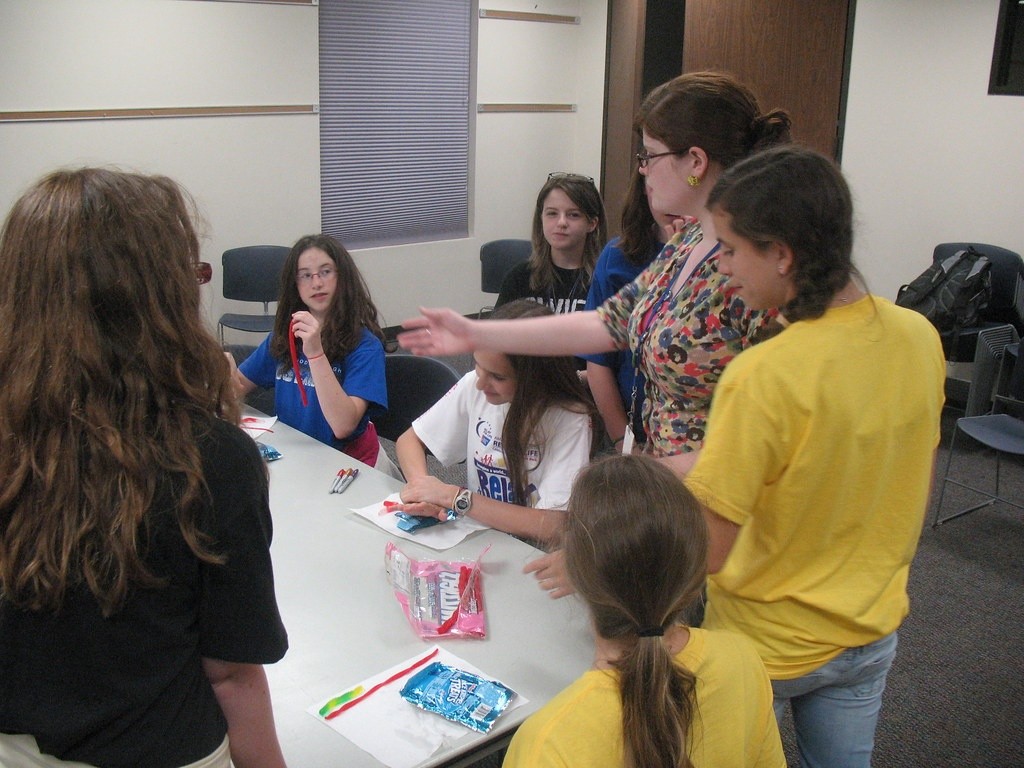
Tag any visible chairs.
[896,241,1024,528]
[217,245,292,352]
[374,354,461,456]
[469,239,533,370]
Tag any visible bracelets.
[611,436,623,448]
[307,352,326,360]
[452,486,467,513]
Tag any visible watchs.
[455,489,474,516]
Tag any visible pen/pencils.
[334,468,353,493]
[337,468,359,493]
[329,469,344,494]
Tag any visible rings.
[426,328,431,335]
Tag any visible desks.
[237,403,595,768]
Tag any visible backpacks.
[892,243,995,365]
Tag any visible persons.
[221,235,405,490]
[0,167,291,768]
[396,71,947,767]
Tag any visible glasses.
[296,268,339,282]
[636,147,689,169]
[546,171,595,184]
[188,261,213,285]
